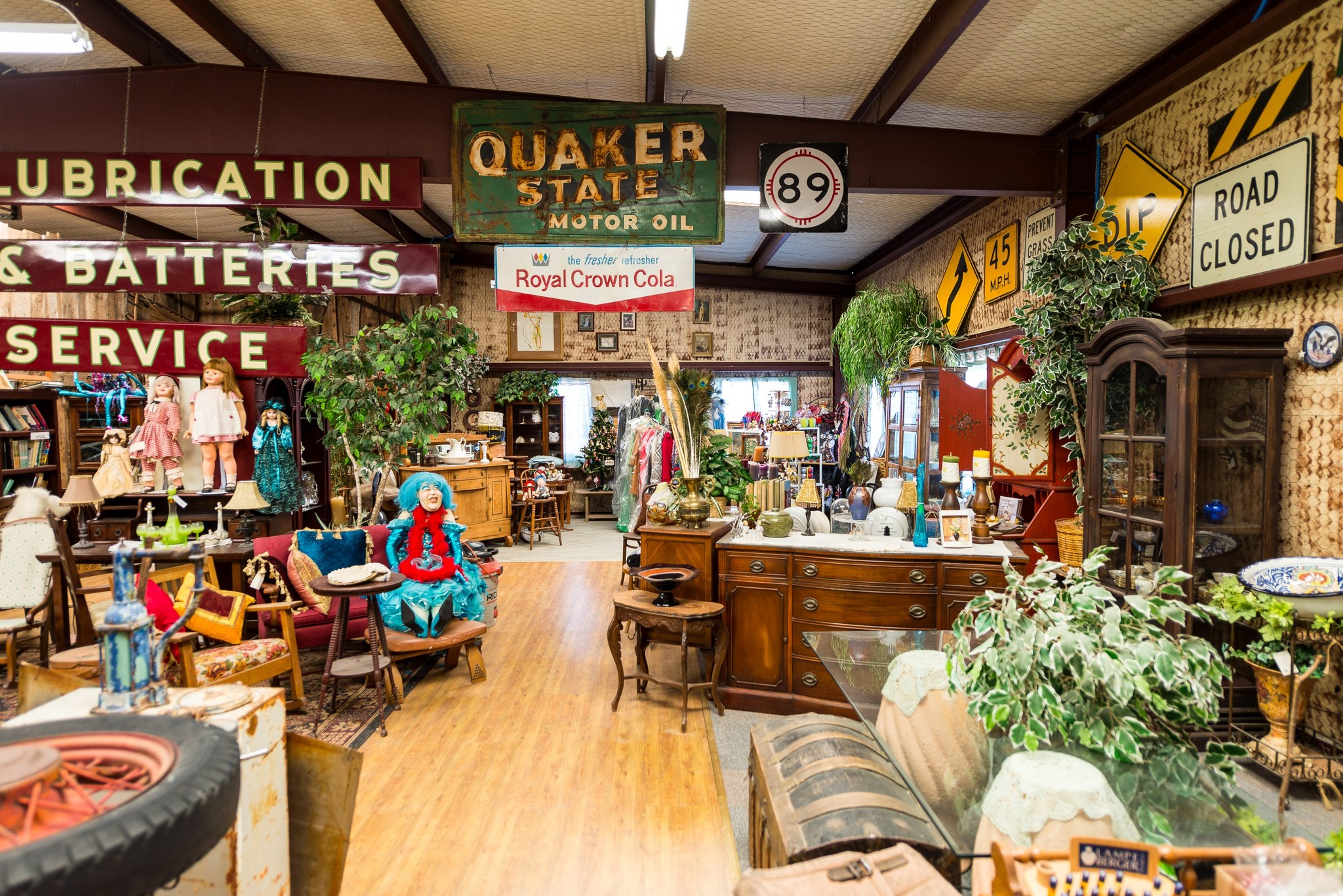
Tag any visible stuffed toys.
[533,462,547,480]
[795,400,833,428]
[545,462,558,478]
[522,481,536,503]
[534,474,551,499]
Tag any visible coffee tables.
[607,590,731,733]
[576,488,618,523]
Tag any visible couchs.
[255,522,392,689]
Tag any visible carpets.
[708,701,794,881]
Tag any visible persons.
[250,396,305,515]
[833,392,847,433]
[809,434,821,462]
[91,428,134,503]
[91,372,135,430]
[127,372,185,494]
[822,431,835,463]
[360,471,490,637]
[183,357,249,493]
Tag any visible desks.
[309,568,407,739]
[802,627,1342,895]
[36,542,258,655]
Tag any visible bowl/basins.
[1238,555,1343,618]
[438,454,474,463]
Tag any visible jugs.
[446,437,467,457]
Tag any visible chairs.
[0,573,54,691]
[106,557,309,721]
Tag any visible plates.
[1108,555,1239,611]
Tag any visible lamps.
[59,474,106,550]
[223,478,273,550]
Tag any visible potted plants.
[1245,639,1329,766]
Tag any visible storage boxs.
[748,712,954,887]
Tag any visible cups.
[809,417,815,427]
[802,418,809,427]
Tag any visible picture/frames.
[505,296,714,362]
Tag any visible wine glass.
[136,523,151,548]
[191,522,205,541]
[159,526,173,549]
[179,523,193,547]
[147,526,160,549]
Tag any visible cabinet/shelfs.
[635,329,1295,735]
[85,376,330,542]
[0,687,294,896]
[398,394,566,550]
[1191,576,1342,814]
[0,388,62,502]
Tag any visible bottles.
[212,501,229,539]
[913,462,929,548]
[142,503,155,549]
[159,479,187,544]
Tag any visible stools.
[362,612,490,706]
[549,491,570,527]
